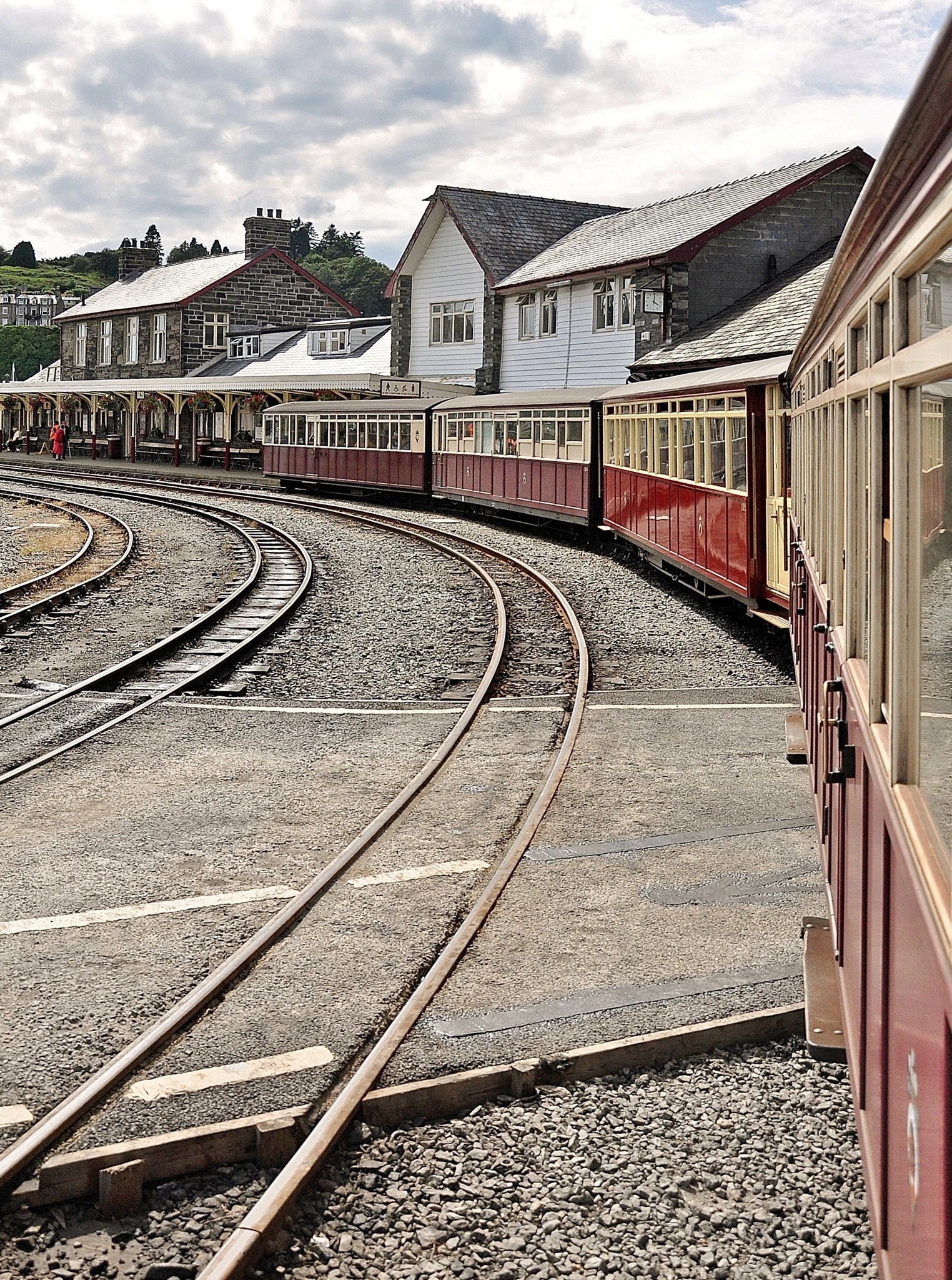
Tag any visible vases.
[34,403,39,408]
[8,403,17,408]
[252,403,266,411]
[148,403,159,410]
[198,400,208,409]
[107,406,116,411]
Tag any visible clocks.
[640,290,666,313]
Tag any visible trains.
[260,3,952,1280]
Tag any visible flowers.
[100,396,125,412]
[141,396,162,412]
[189,392,217,412]
[242,395,267,413]
[28,397,46,408]
[60,397,76,411]
[316,390,337,402]
[2,397,18,411]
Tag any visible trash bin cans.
[196,438,212,466]
[107,434,121,459]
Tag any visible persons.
[49,420,70,461]
[9,428,22,452]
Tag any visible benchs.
[132,440,261,471]
[8,432,111,458]
